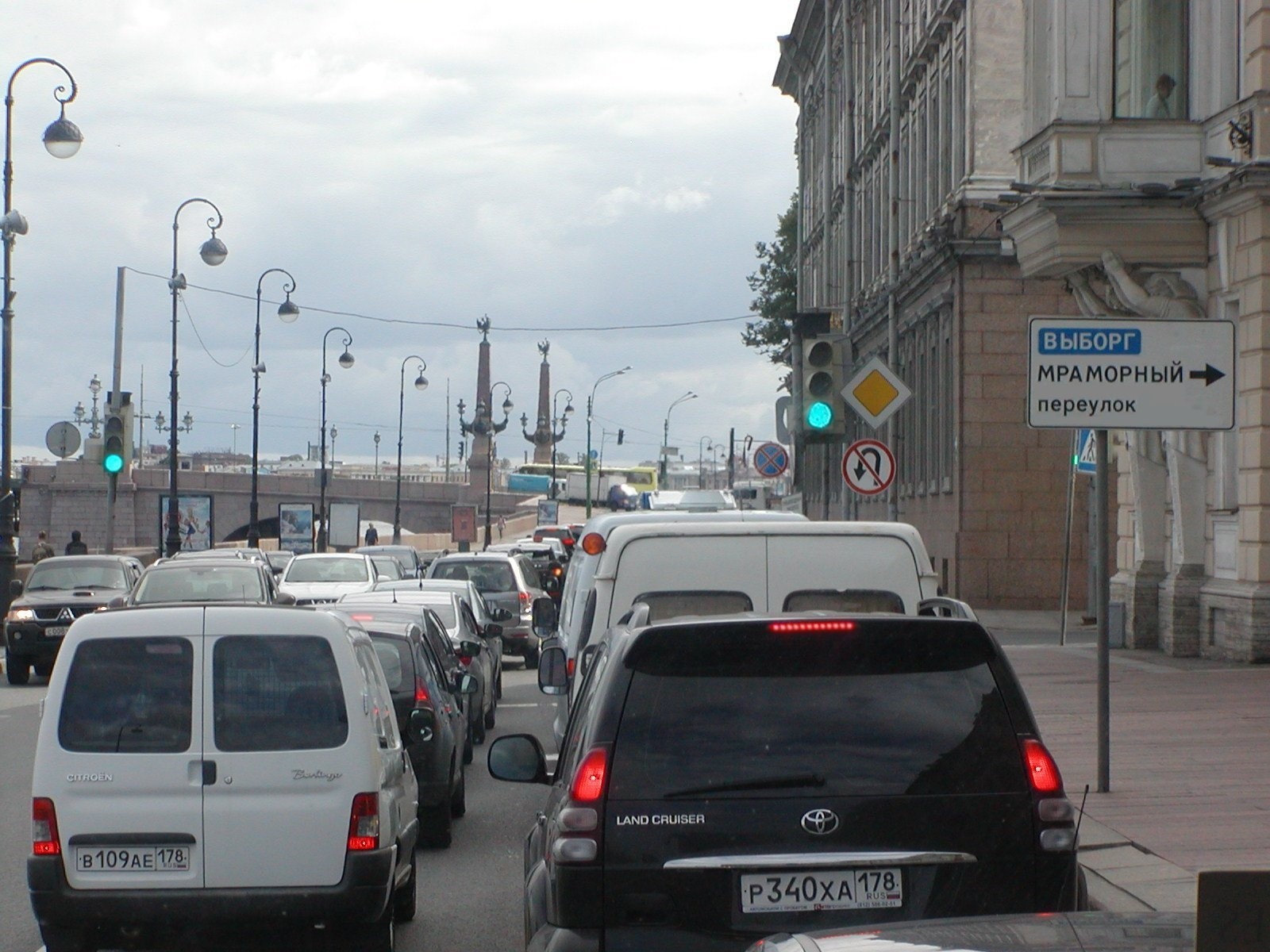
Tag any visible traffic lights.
[102,414,126,473]
[802,338,848,435]
[618,428,624,444]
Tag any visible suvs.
[421,523,582,670]
[485,592,1088,951]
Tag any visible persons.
[32,531,54,566]
[453,567,469,581]
[496,514,506,539]
[365,522,378,546]
[65,530,88,556]
[164,506,210,549]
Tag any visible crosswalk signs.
[1075,427,1099,474]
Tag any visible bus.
[515,462,660,495]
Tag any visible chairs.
[285,685,332,720]
[453,565,469,580]
[207,583,227,591]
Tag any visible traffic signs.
[1027,314,1236,431]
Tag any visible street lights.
[663,390,699,492]
[698,436,728,491]
[247,266,300,548]
[0,56,84,645]
[156,407,194,466]
[74,371,104,464]
[392,353,430,546]
[317,326,355,554]
[372,430,381,475]
[585,365,635,518]
[165,197,230,558]
[470,380,575,549]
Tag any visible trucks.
[506,471,640,512]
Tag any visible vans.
[531,508,942,752]
[24,602,435,951]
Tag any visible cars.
[4,544,505,850]
